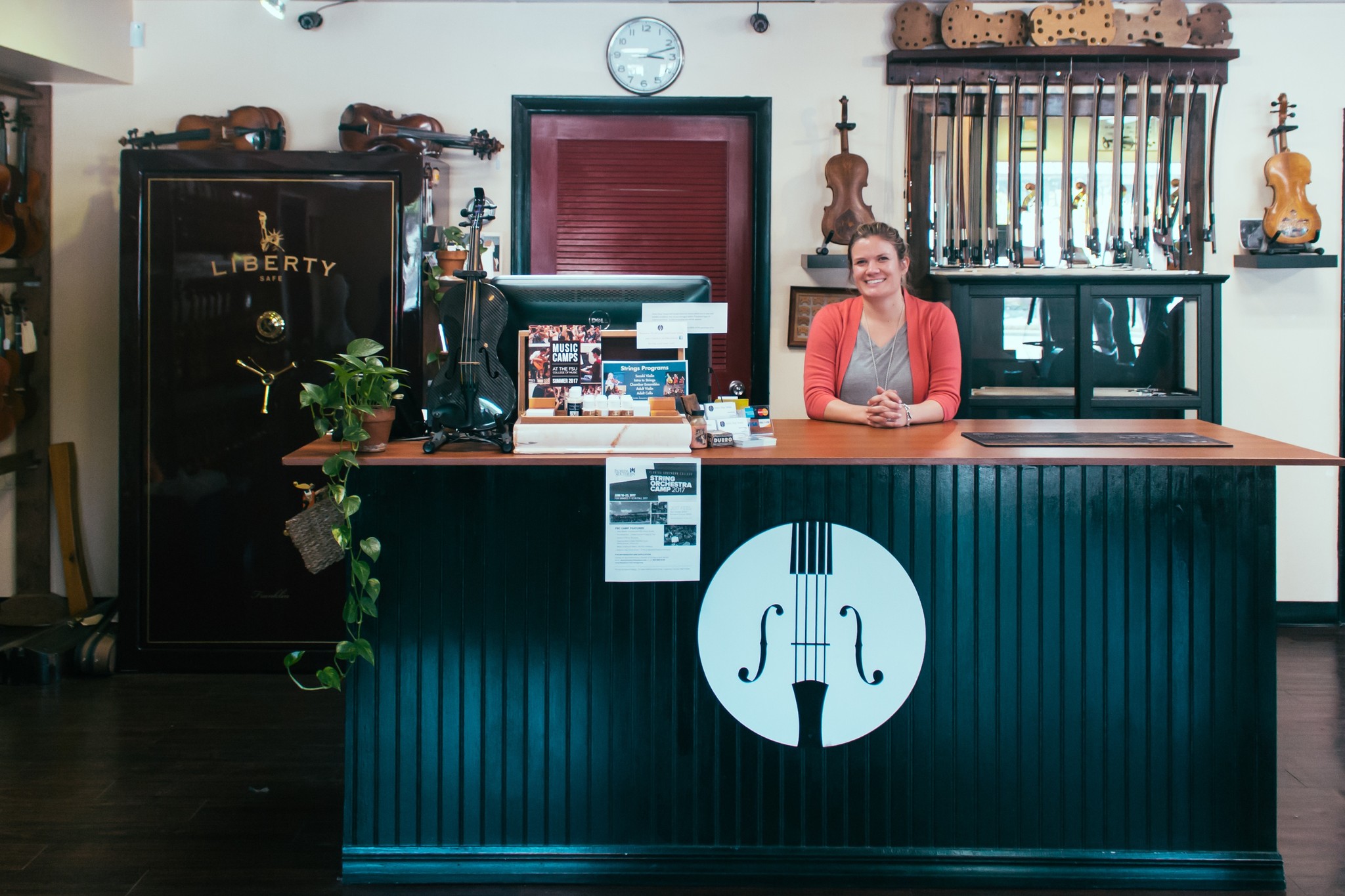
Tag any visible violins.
[820,94,877,245]
[0,291,39,444]
[425,184,519,434]
[118,107,285,157]
[337,101,505,161]
[0,101,45,260]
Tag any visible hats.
[591,325,594,328]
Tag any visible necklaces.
[860,298,905,391]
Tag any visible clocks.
[606,17,685,95]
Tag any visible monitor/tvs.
[490,273,713,408]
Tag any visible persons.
[605,372,620,394]
[532,384,602,411]
[803,222,961,428]
[581,347,601,383]
[665,372,685,396]
[528,325,602,343]
[529,348,550,383]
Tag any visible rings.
[886,417,891,421]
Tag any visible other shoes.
[535,380,538,383]
[543,375,549,379]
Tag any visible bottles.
[567,385,635,418]
[689,409,707,448]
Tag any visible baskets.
[285,482,346,575]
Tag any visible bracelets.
[901,403,911,426]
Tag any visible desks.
[287,413,1345,896]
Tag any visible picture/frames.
[787,286,861,348]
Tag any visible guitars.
[1260,92,1322,244]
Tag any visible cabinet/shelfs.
[116,149,449,670]
[0,74,68,629]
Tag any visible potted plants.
[435,226,488,276]
[283,338,410,691]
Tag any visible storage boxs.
[513,330,692,454]
